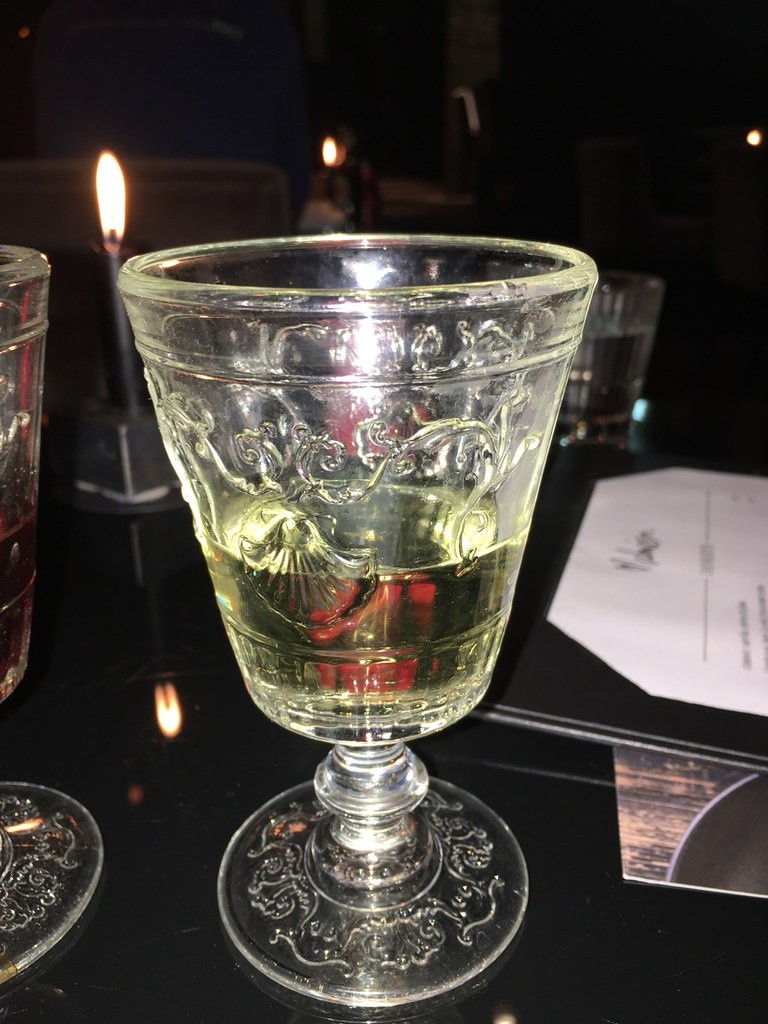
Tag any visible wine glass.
[0,246,103,981]
[119,234,599,1012]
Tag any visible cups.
[552,266,665,444]
[0,158,287,504]
